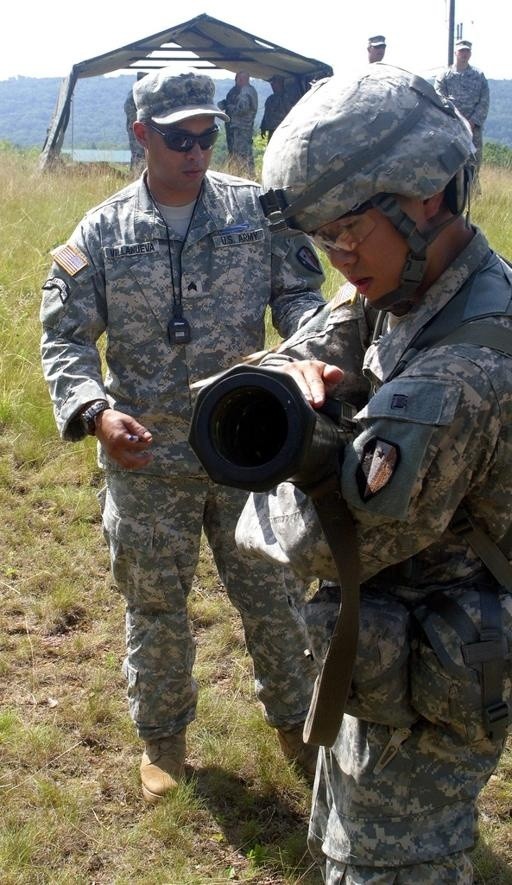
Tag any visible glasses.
[303,214,376,253]
[147,123,218,152]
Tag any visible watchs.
[80,400,109,436]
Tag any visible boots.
[275,721,319,777]
[140,727,186,802]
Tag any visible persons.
[36,67,333,804]
[233,62,511,884]
[219,70,294,180]
[123,71,149,172]
[435,39,490,197]
[366,35,387,65]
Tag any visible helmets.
[259,63,473,234]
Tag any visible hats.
[456,40,472,50]
[368,36,386,46]
[133,65,230,125]
